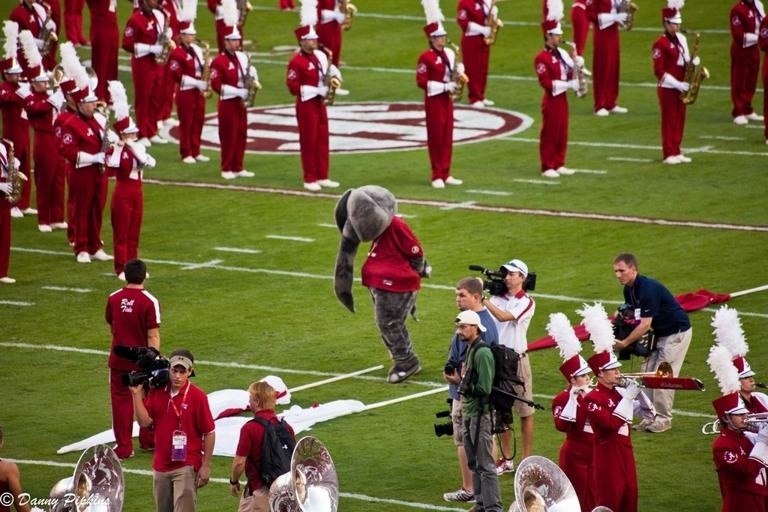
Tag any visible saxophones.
[446,39,468,101]
[620,0,638,30]
[194,38,214,98]
[320,41,340,105]
[93,106,110,173]
[37,1,58,56]
[0,138,28,204]
[154,6,176,64]
[484,0,504,44]
[559,40,591,97]
[678,29,710,103]
[236,47,262,107]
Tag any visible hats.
[169,356,193,370]
[456,309,487,332]
[423,21,448,37]
[733,357,756,378]
[587,350,622,375]
[662,8,682,24]
[559,354,593,379]
[541,20,563,35]
[179,20,197,35]
[295,25,319,40]
[712,392,750,418]
[0,58,98,104]
[224,24,242,40]
[500,259,528,278]
[113,117,140,134]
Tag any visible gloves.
[748,417,768,468]
[612,379,656,424]
[248,66,258,80]
[465,6,498,38]
[215,5,240,21]
[320,9,345,25]
[221,84,249,100]
[551,56,584,96]
[427,63,465,97]
[743,32,759,48]
[46,87,64,112]
[7,157,20,170]
[34,19,56,50]
[76,128,157,169]
[134,42,163,58]
[598,12,628,31]
[0,182,13,196]
[180,74,207,91]
[559,386,593,423]
[657,56,701,93]
[166,27,173,40]
[300,64,342,102]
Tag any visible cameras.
[445,362,457,374]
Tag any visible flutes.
[118,138,138,146]
[574,385,591,392]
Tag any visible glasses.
[506,262,526,273]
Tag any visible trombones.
[589,361,704,389]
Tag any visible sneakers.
[541,167,576,177]
[1,276,16,283]
[75,41,90,47]
[663,154,692,164]
[303,179,340,192]
[443,487,483,512]
[10,205,69,233]
[136,117,181,147]
[644,420,672,432]
[496,457,514,476]
[221,169,255,179]
[733,112,765,125]
[593,105,628,116]
[76,247,149,280]
[582,68,592,76]
[335,87,349,96]
[182,154,210,164]
[431,176,463,189]
[472,98,495,108]
[632,419,654,431]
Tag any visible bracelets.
[230,478,238,485]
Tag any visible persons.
[0,426,30,511]
[755,11,767,140]
[613,253,692,434]
[2,1,635,282]
[105,259,161,459]
[727,1,763,125]
[332,185,434,384]
[441,287,768,512]
[128,349,215,512]
[649,0,700,166]
[230,382,296,512]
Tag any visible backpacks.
[472,341,518,412]
[248,416,296,488]
